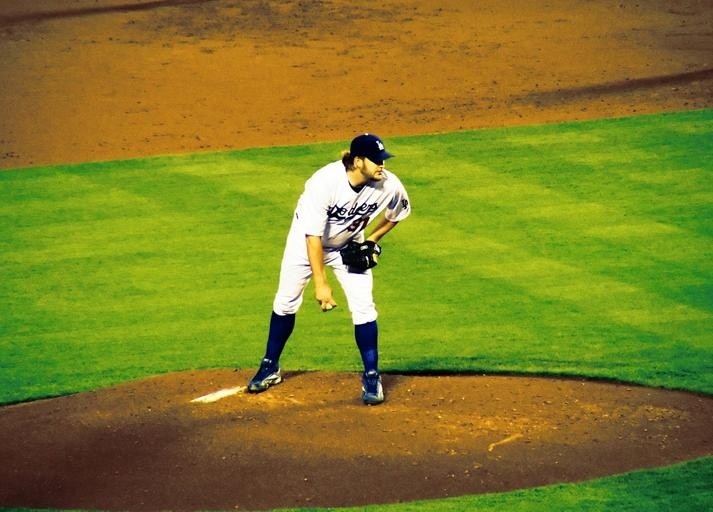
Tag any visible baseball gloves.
[340,240,381,273]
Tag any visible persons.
[244,131,411,407]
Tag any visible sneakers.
[362,368,385,406]
[247,357,283,392]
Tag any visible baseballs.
[325,303,333,310]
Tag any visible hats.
[350,132,396,163]
